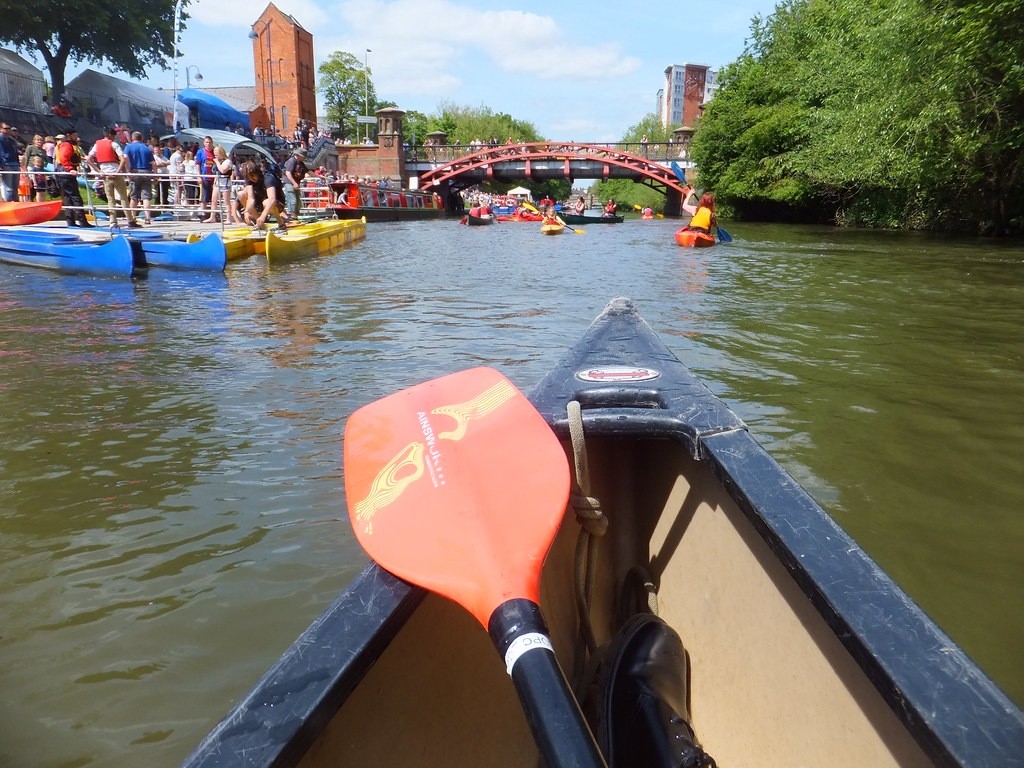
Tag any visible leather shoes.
[598,613,717,768]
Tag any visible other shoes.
[270,224,287,234]
[80,222,96,229]
[128,221,142,228]
[68,224,81,229]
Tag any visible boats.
[496,214,543,221]
[179,295,1024,768]
[307,180,444,222]
[0,198,64,226]
[674,226,715,247]
[558,211,625,224]
[467,213,493,226]
[186,215,366,264]
[0,226,230,279]
[541,225,565,235]
[640,215,655,220]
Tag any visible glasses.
[3,128,10,129]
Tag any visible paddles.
[522,203,584,233]
[634,204,665,218]
[340,366,613,768]
[671,160,733,242]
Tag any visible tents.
[0,49,45,113]
[177,86,250,138]
[508,186,531,201]
[64,69,190,131]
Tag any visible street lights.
[364,48,373,137]
[248,20,276,137]
[186,64,203,88]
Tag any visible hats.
[294,147,308,158]
[11,127,18,131]
[55,135,66,140]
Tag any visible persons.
[468,186,472,192]
[283,147,324,220]
[0,122,234,231]
[602,613,717,768]
[41,96,49,115]
[314,166,389,208]
[402,136,526,160]
[475,187,479,194]
[642,205,654,216]
[360,138,374,145]
[469,202,493,218]
[639,135,647,156]
[545,139,574,152]
[566,196,585,215]
[232,150,287,230]
[678,143,686,159]
[601,198,617,217]
[293,124,351,150]
[683,188,719,234]
[253,125,280,136]
[59,101,72,116]
[543,200,555,225]
[666,135,674,159]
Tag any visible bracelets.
[243,210,251,214]
